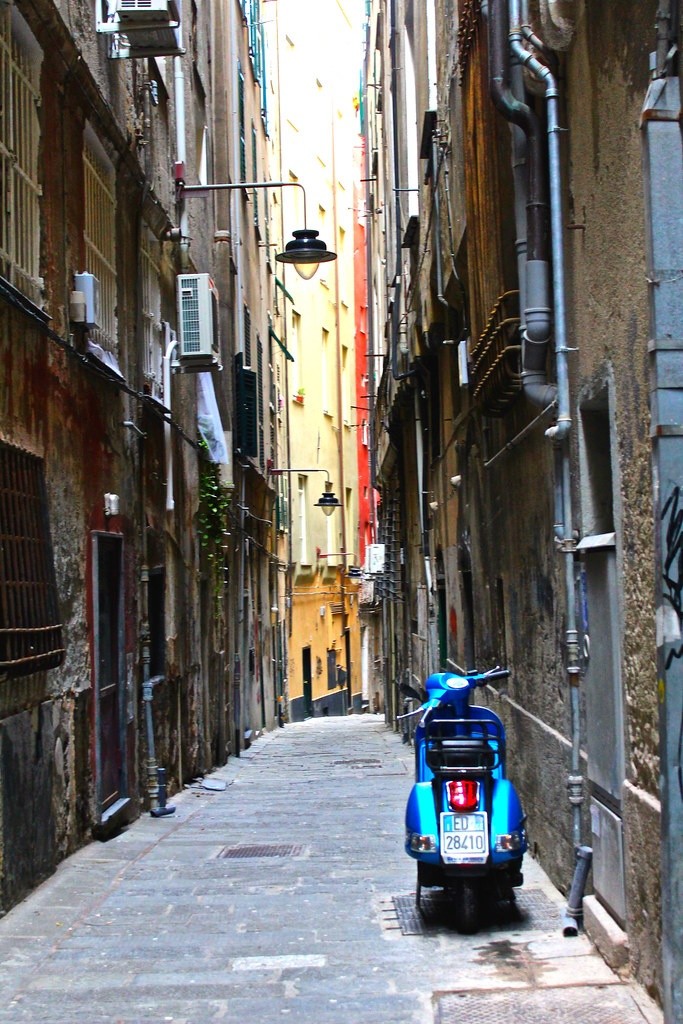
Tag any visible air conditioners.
[115,0,183,50]
[175,273,222,367]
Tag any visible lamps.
[319,552,364,584]
[184,182,338,281]
[272,469,344,517]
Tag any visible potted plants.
[297,388,306,403]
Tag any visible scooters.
[393,665,529,936]
